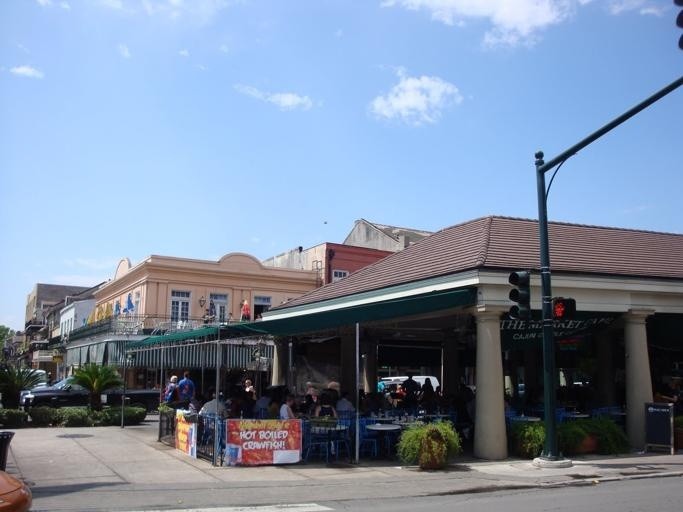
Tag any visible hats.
[171,376,178,383]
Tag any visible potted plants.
[0,360,125,412]
[674,414,683,450]
[557,416,630,457]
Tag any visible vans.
[3,368,47,388]
[379,375,440,399]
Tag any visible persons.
[228,313,234,320]
[161,372,357,420]
[203,309,211,324]
[504,376,682,415]
[358,371,477,428]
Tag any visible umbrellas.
[240,300,250,322]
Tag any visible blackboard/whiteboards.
[643,402,674,448]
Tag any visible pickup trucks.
[19,375,160,411]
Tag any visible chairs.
[200,407,451,467]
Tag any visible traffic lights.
[507,270,532,323]
[552,297,577,321]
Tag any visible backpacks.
[164,384,177,402]
